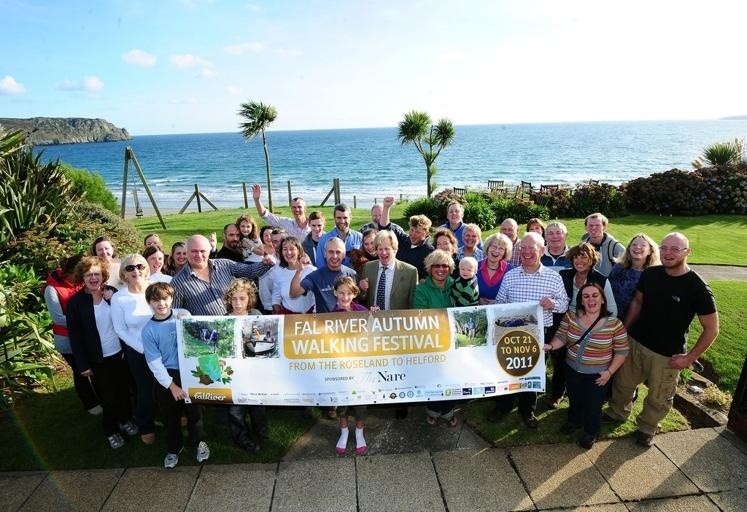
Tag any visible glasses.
[228,232,241,236]
[123,264,145,272]
[335,217,347,219]
[431,265,449,268]
[659,246,688,253]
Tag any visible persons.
[601,231,721,448]
[41,184,666,472]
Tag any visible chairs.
[453,179,559,200]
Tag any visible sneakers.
[87,404,210,469]
[425,394,656,450]
[327,406,337,420]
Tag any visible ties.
[376,267,388,311]
